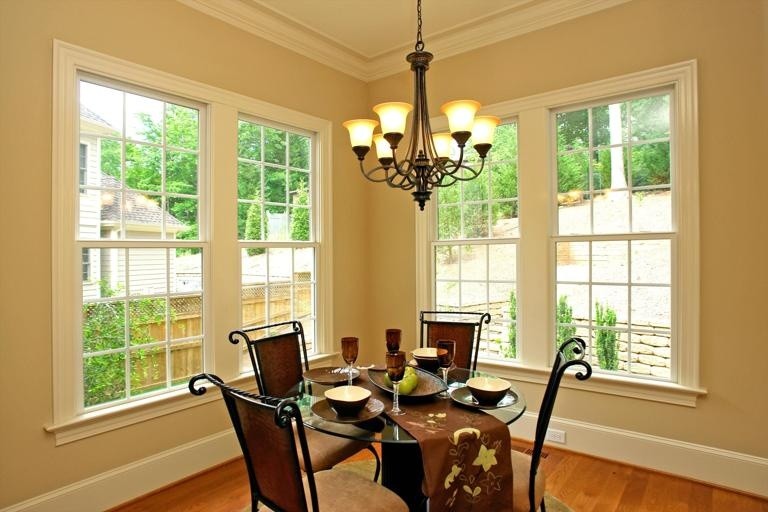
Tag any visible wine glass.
[341,337,359,386]
[437,340,456,397]
[385,351,406,416]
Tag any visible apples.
[384,366,418,395]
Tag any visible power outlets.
[544,429,565,444]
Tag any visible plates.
[311,398,385,423]
[409,358,457,377]
[449,386,520,410]
[300,366,360,385]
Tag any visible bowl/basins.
[466,377,511,405]
[412,347,448,371]
[323,386,372,415]
[365,362,448,397]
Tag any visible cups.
[385,328,401,354]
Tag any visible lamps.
[343,2,500,214]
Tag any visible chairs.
[226,320,380,483]
[420,335,593,512]
[187,366,412,511]
[417,309,490,373]
